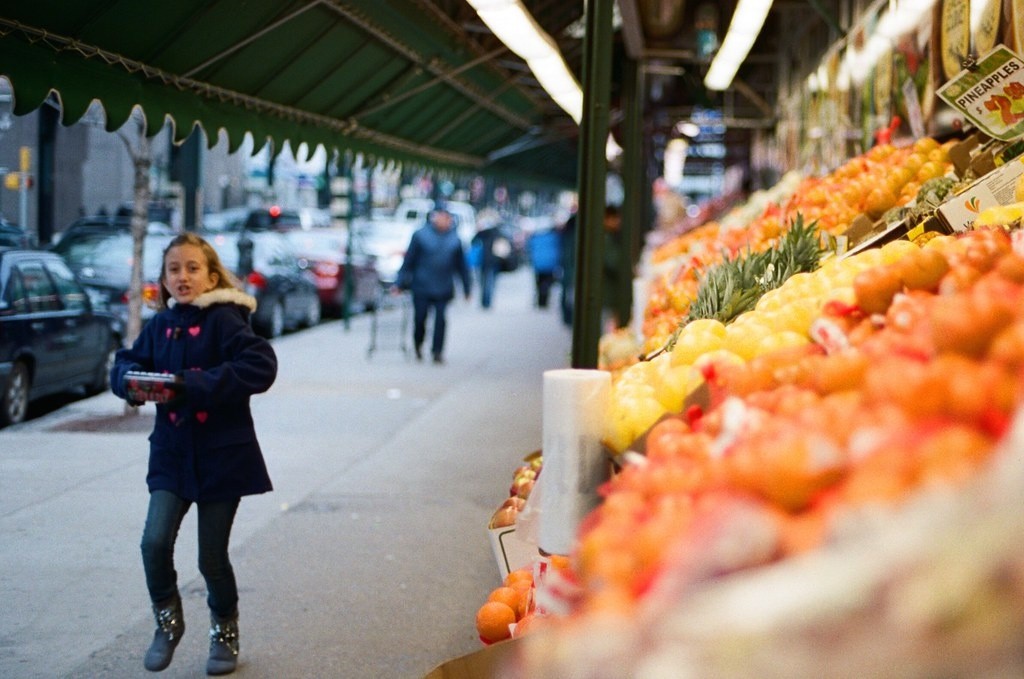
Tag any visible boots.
[206,611,240,675]
[144,600,185,672]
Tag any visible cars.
[0,245,128,425]
[48,197,476,341]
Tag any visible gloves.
[156,382,187,428]
[122,363,144,406]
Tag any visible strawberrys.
[130,379,173,402]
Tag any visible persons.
[390,191,620,362]
[111,233,278,675]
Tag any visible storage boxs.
[423,629,538,679]
[488,507,538,581]
[846,130,1024,257]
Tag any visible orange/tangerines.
[476,136,1024,642]
